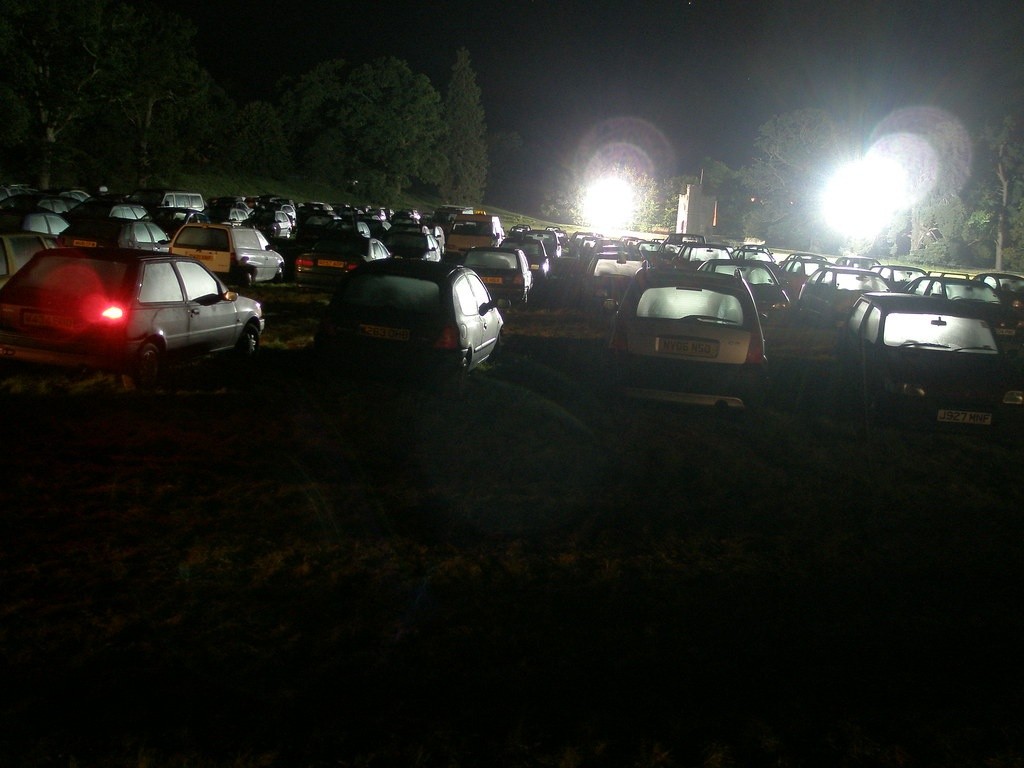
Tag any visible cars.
[568,230,1024,434]
[313,223,567,388]
[0,180,500,392]
